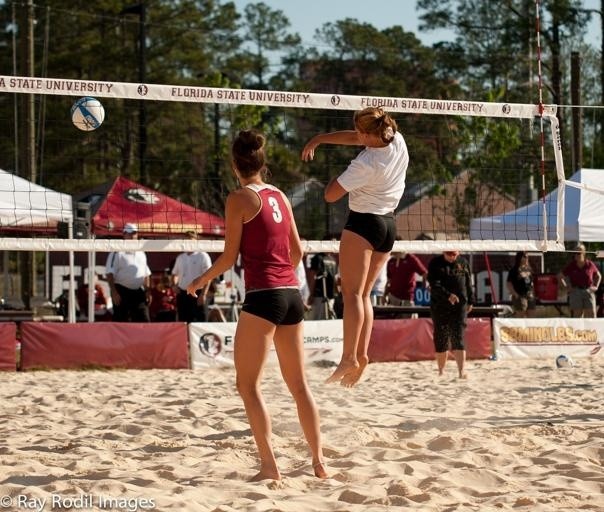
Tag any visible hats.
[124,223,139,233]
[185,230,198,239]
[446,251,459,256]
[573,245,586,255]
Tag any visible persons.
[506,252,535,318]
[556,244,602,318]
[293,258,313,312]
[426,251,475,378]
[314,237,338,315]
[186,131,347,483]
[150,274,177,322]
[300,106,411,388]
[168,231,213,323]
[372,234,430,308]
[105,221,154,323]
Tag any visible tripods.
[315,277,337,320]
[225,293,239,322]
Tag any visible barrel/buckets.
[534,272,557,301]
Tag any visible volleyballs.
[557,355,569,368]
[70,97,106,130]
